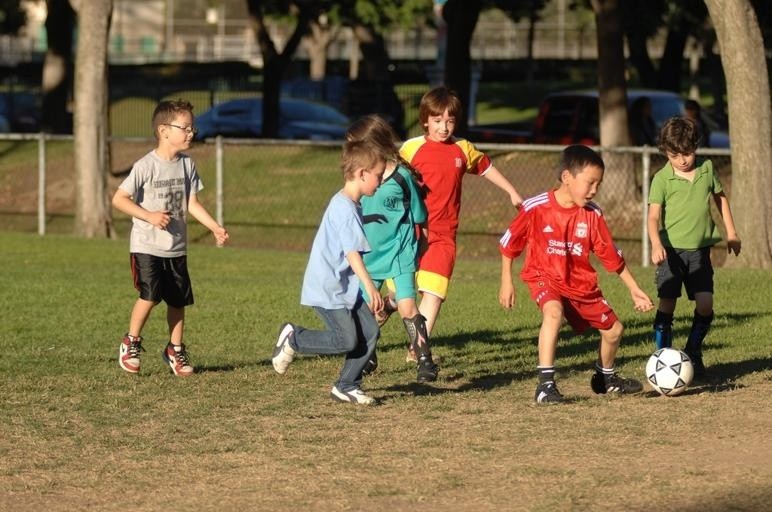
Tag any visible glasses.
[163,122,199,134]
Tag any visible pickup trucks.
[467,84,732,148]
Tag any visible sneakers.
[589,371,643,395]
[353,351,379,384]
[684,349,706,380]
[118,332,144,374]
[533,384,567,404]
[161,340,194,378]
[271,321,299,376]
[330,385,376,406]
[408,352,443,383]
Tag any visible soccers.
[646,348,693,396]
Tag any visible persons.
[681,102,710,145]
[369,86,525,365]
[112,98,230,378]
[627,95,658,145]
[646,116,742,375]
[270,138,385,406]
[343,116,439,381]
[498,144,656,404]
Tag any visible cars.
[191,97,356,141]
